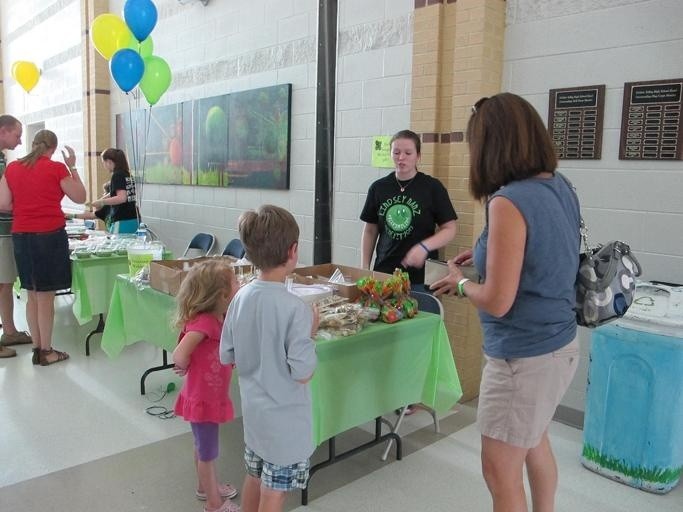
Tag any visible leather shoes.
[0,343,17,357]
[1,331,32,345]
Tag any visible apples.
[206,106,226,145]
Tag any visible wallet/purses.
[424,259,477,297]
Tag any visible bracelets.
[458,278,467,297]
[100,199,104,209]
[419,241,429,253]
[69,213,77,219]
[66,165,78,172]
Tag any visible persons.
[91,148,139,233]
[217,202,319,512]
[0,130,86,366]
[427,92,583,512]
[359,130,458,415]
[61,181,112,232]
[171,259,243,512]
[0,114,31,361]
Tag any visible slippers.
[204,499,240,512]
[196,483,237,502]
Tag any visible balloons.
[85,14,128,60]
[10,60,42,96]
[123,1,157,44]
[126,33,154,62]
[138,54,173,108]
[107,48,145,95]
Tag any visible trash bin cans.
[580,282,683,494]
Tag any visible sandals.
[32,346,42,365]
[40,347,69,365]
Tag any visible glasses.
[470,96,489,115]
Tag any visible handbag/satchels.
[575,240,642,327]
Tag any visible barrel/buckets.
[127,242,163,281]
